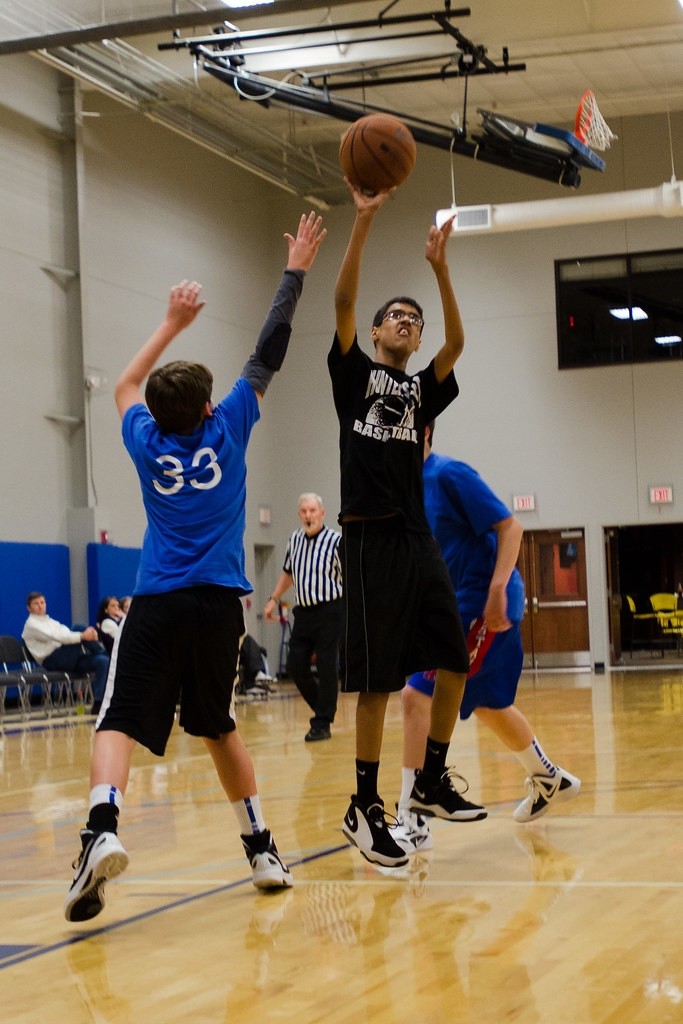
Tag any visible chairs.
[0,635,94,729]
[625,592,683,661]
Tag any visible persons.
[239,634,273,695]
[95,596,125,656]
[63,209,327,920]
[21,592,109,715]
[328,176,487,869]
[263,494,343,741]
[119,596,132,612]
[387,418,579,857]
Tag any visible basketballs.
[340,116,416,191]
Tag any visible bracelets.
[266,596,277,604]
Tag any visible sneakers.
[240,829,293,888]
[513,765,581,824]
[388,802,434,855]
[408,765,488,823]
[341,794,410,867]
[63,822,130,922]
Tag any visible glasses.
[380,311,424,338]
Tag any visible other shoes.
[256,671,278,685]
[246,687,266,693]
[305,727,332,740]
